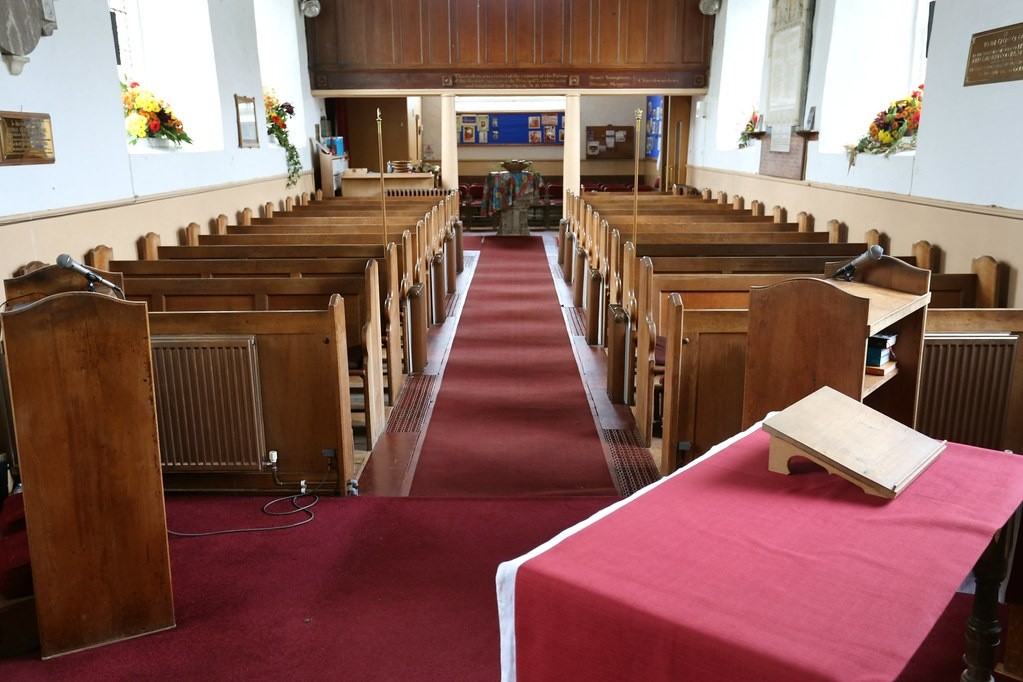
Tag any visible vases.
[502,164,528,171]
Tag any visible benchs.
[559,184,1023,476]
[19,190,464,495]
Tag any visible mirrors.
[234,94,260,149]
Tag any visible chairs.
[459,177,660,231]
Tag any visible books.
[866,335,896,376]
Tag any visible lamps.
[299,0,320,17]
[699,0,720,15]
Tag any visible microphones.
[57,254,121,292]
[833,245,883,277]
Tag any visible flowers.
[265,91,302,190]
[739,111,758,149]
[847,84,924,172]
[120,74,193,147]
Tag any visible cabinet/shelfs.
[319,148,349,197]
[1,264,176,660]
[741,254,932,432]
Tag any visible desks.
[496,413,1023,682]
[481,171,545,236]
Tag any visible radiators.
[151,333,267,474]
[916,330,1018,452]
